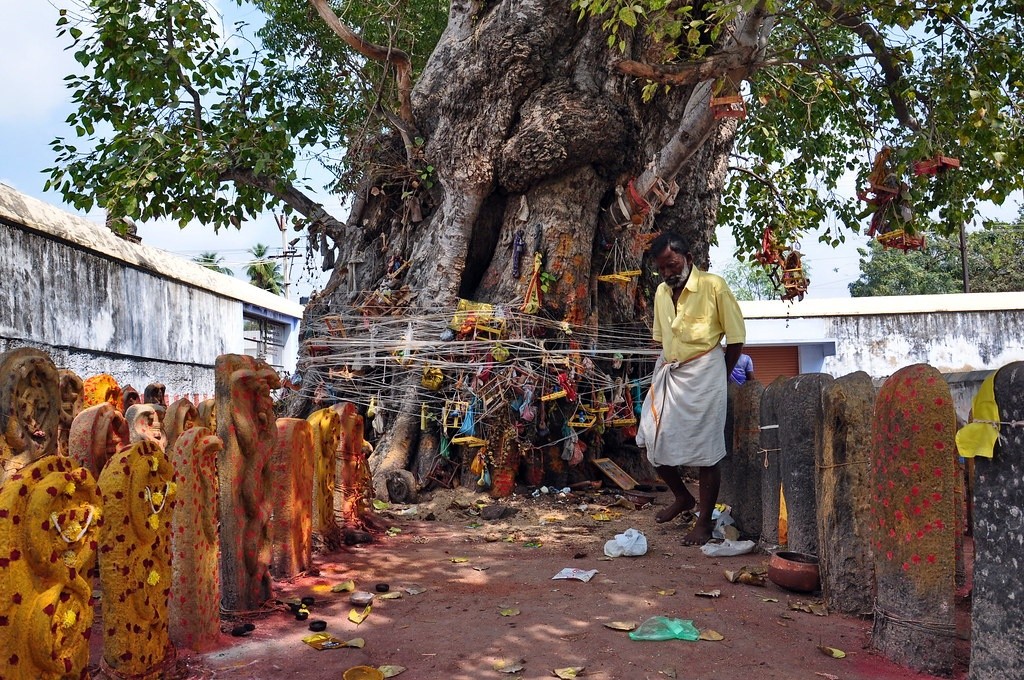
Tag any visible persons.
[724,347,755,385]
[636,233,746,547]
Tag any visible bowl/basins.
[623,489,657,508]
[767,550,820,590]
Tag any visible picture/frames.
[591,457,640,491]
